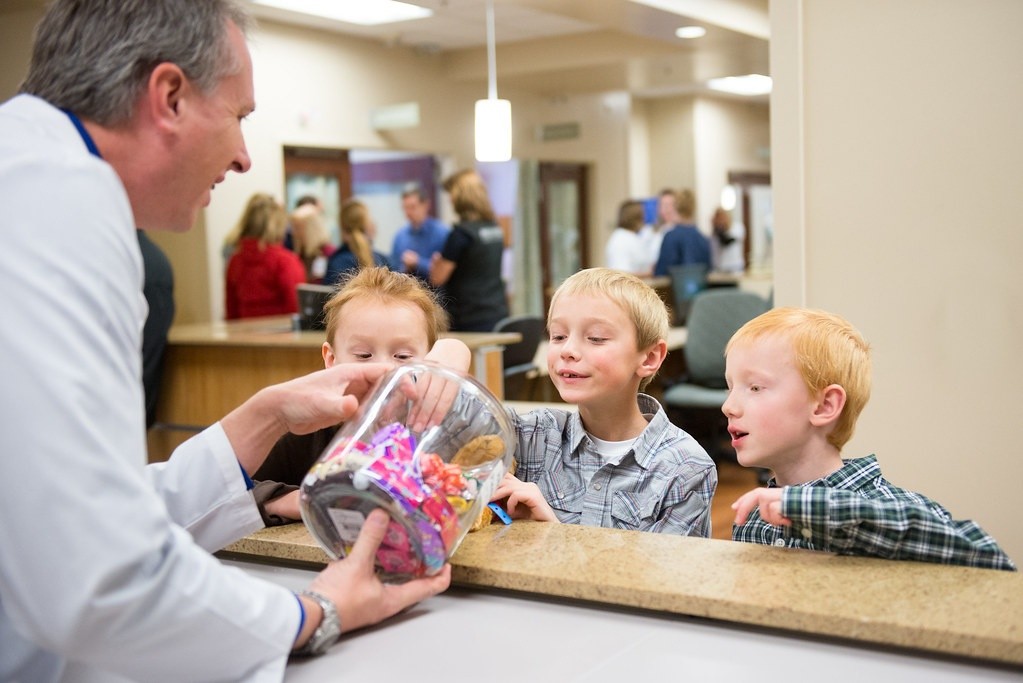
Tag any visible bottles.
[300,361,515,582]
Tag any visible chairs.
[662,289,773,478]
[494,315,545,401]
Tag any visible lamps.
[475,0,512,163]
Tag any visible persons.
[0,0,452,683]
[722,308,1017,572]
[134,221,178,434]
[408,268,718,539]
[224,162,519,330]
[606,186,743,324]
[250,261,461,528]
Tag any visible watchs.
[296,589,340,657]
